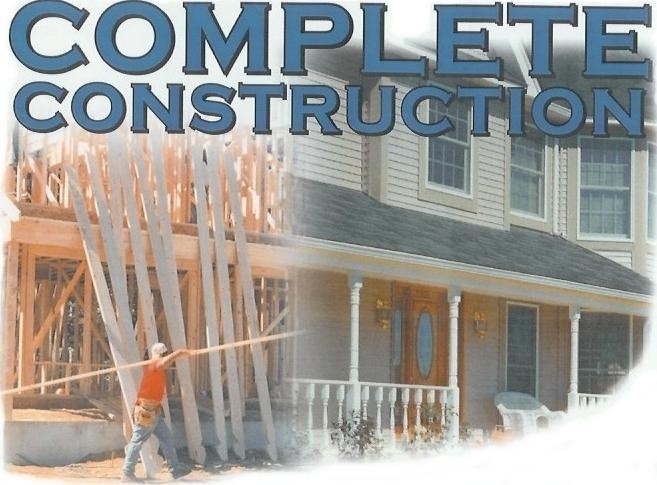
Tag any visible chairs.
[493,391,567,435]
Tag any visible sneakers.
[122,475,140,483]
[171,468,190,480]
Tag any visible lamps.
[474,310,488,339]
[375,300,393,328]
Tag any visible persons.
[119,343,192,483]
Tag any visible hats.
[151,343,168,359]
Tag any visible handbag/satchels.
[133,402,156,427]
